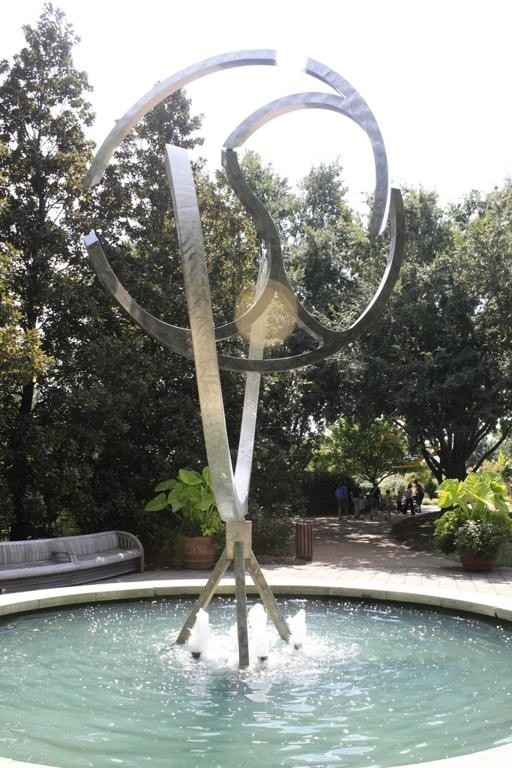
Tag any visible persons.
[333,476,425,520]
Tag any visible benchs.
[1,529,145,595]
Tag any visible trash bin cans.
[295,520,313,562]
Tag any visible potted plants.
[432,465,512,574]
[144,466,226,570]
